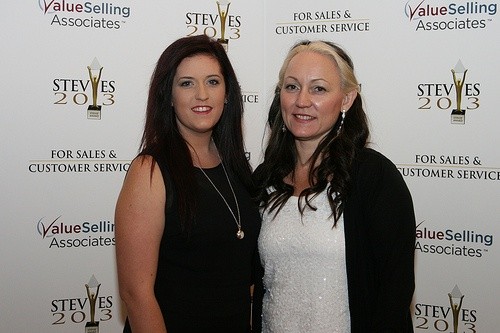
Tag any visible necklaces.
[191,138,244,240]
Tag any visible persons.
[247,41,416,333]
[114,35,263,333]
